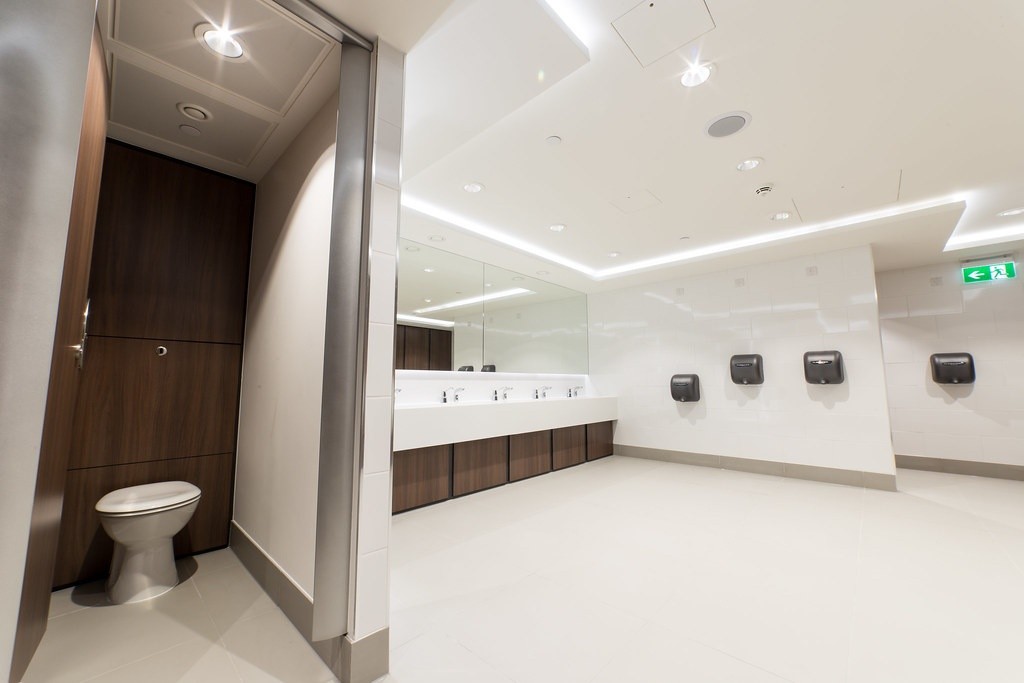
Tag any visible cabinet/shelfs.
[392,420,615,514]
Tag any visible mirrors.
[394,237,590,376]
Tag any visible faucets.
[442,386,465,403]
[535,386,552,400]
[494,385,514,401]
[568,385,583,398]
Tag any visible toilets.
[94,480,202,604]
